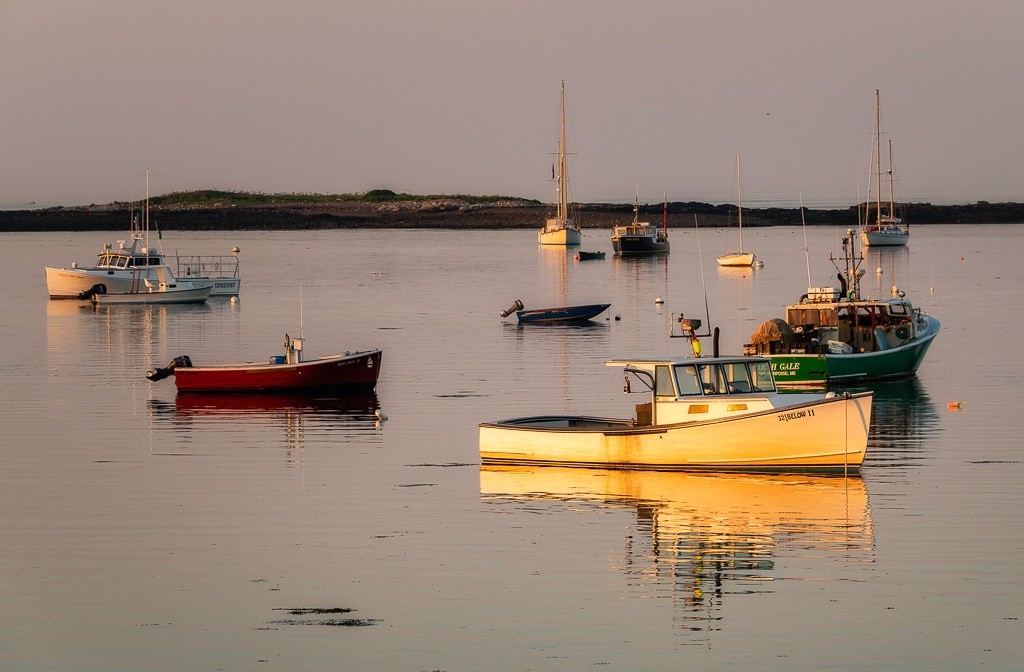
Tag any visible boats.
[499,299,612,323]
[477,210,874,476]
[578,250,606,261]
[478,462,877,652]
[739,194,943,389]
[42,200,243,301]
[146,389,387,471]
[146,282,384,396]
[609,184,671,257]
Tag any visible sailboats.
[715,148,757,268]
[856,88,911,248]
[536,76,583,245]
[76,168,214,307]
